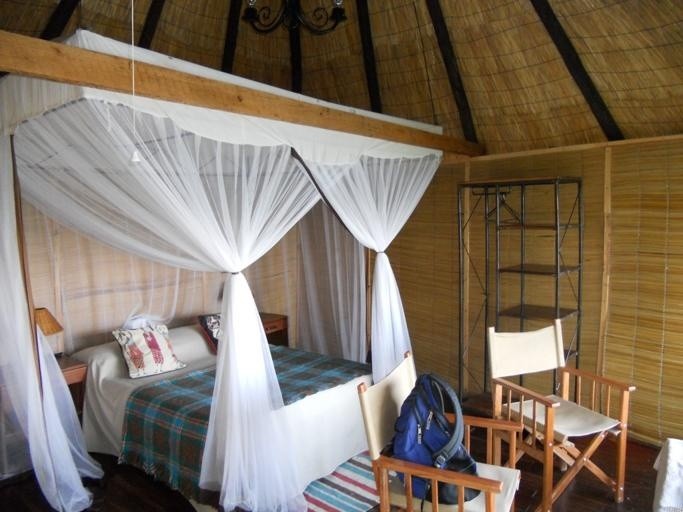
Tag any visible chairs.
[487,318,636,511]
[358,348,522,512]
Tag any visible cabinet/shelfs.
[457,177,582,417]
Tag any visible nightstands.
[0,352,86,477]
[259,312,289,346]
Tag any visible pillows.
[194,312,220,355]
[111,324,187,378]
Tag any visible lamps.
[34,308,64,339]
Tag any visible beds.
[71,322,372,512]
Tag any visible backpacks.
[377,374,482,506]
